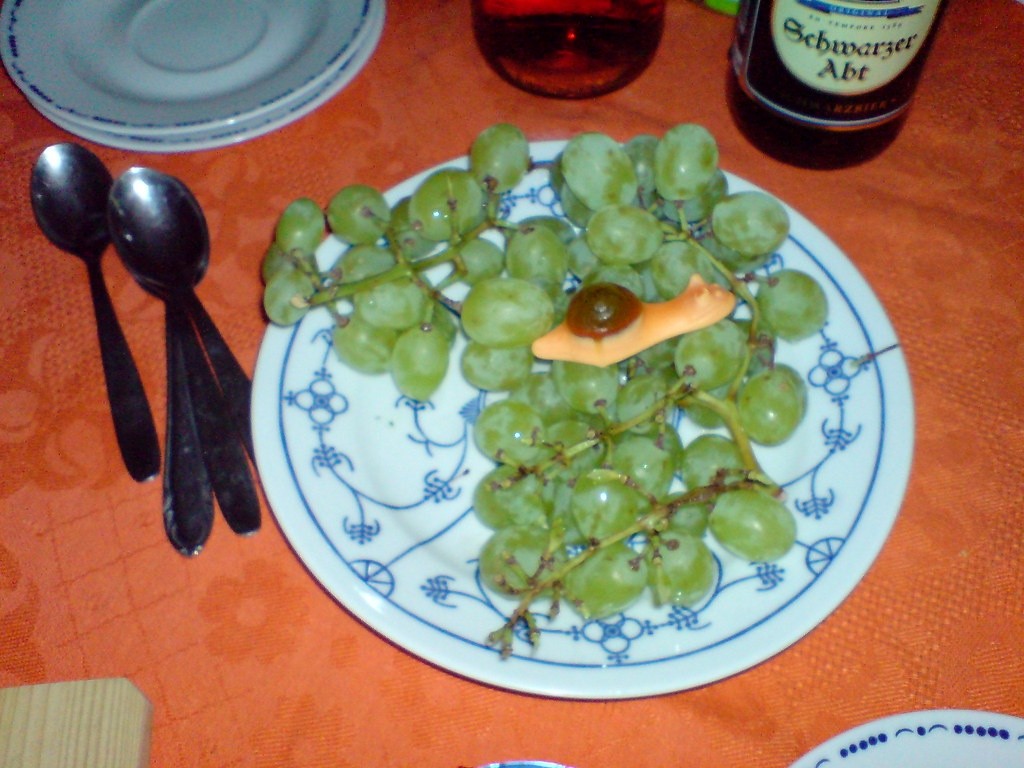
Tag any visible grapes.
[262,122,827,619]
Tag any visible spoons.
[106,166,262,558]
[28,141,161,485]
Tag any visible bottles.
[468,0,667,100]
[725,0,949,171]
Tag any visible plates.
[786,709,1024,768]
[251,138,916,701]
[0,0,386,154]
